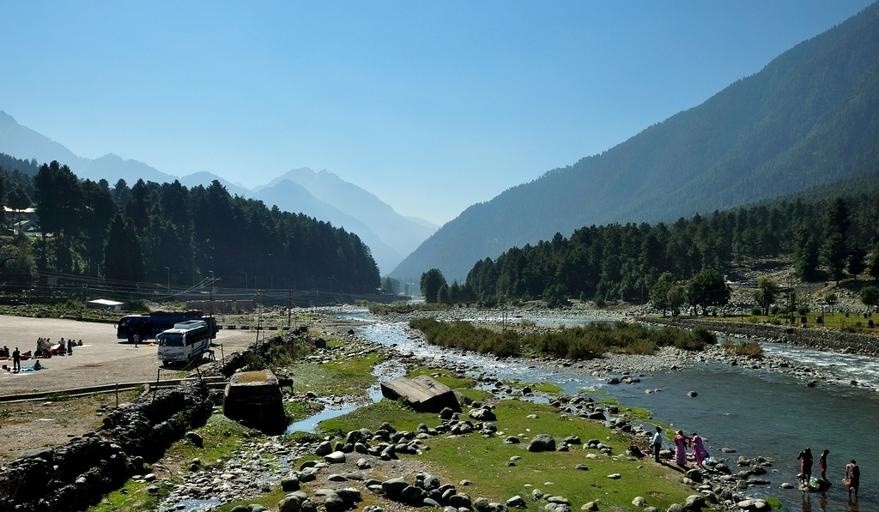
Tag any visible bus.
[114,311,218,368]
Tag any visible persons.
[0,346,10,359]
[649,426,663,463]
[819,448,829,481]
[844,459,860,498]
[797,447,814,489]
[21,351,32,356]
[675,430,706,466]
[33,360,42,370]
[133,332,140,347]
[799,491,829,512]
[845,498,859,512]
[37,336,83,358]
[11,346,21,373]
